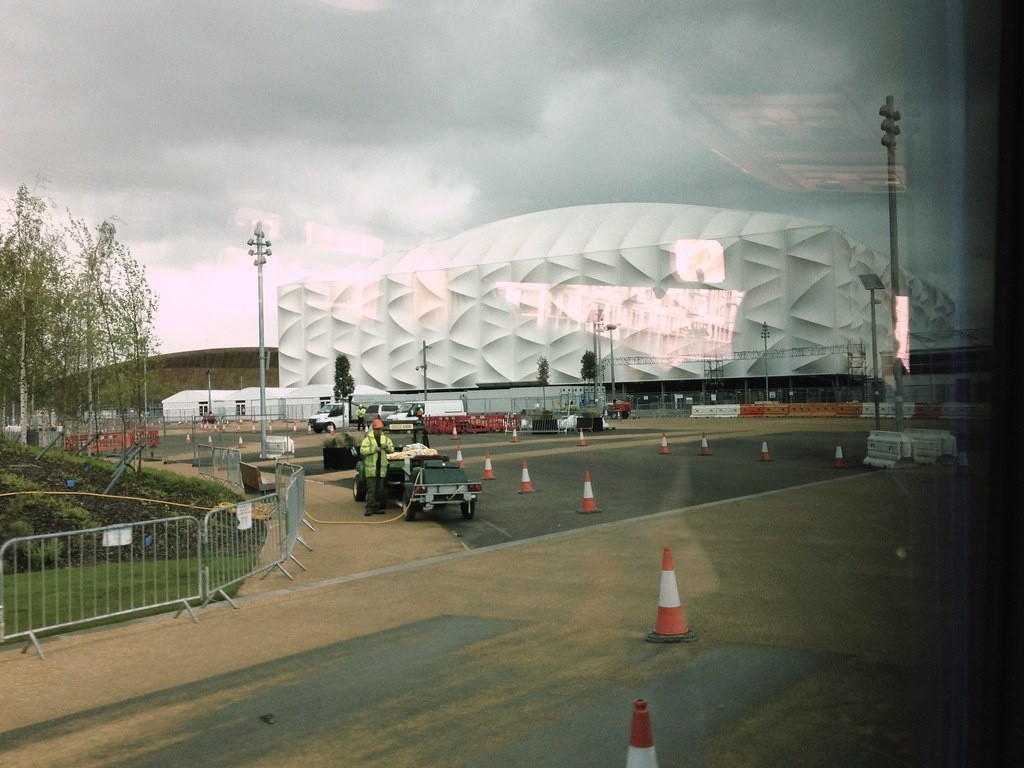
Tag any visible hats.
[359,404,362,406]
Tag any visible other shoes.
[372,509,385,514]
[365,511,371,516]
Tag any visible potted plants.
[577,350,605,432]
[323,354,362,467]
[532,355,558,434]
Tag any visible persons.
[360,420,394,516]
[356,404,366,431]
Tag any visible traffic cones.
[450,423,519,480]
[658,432,672,455]
[644,547,699,643]
[616,411,622,419]
[696,432,713,456]
[756,438,773,463]
[517,460,536,493]
[625,699,661,768]
[575,427,589,446]
[577,471,603,514]
[831,442,849,469]
[159,418,335,450]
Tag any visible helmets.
[373,418,384,429]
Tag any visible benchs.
[239,462,287,502]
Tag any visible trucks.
[308,402,365,434]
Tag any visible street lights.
[859,272,885,430]
[878,103,908,431]
[586,303,617,408]
[247,228,273,461]
[416,365,427,400]
[762,323,770,402]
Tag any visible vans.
[362,400,468,422]
